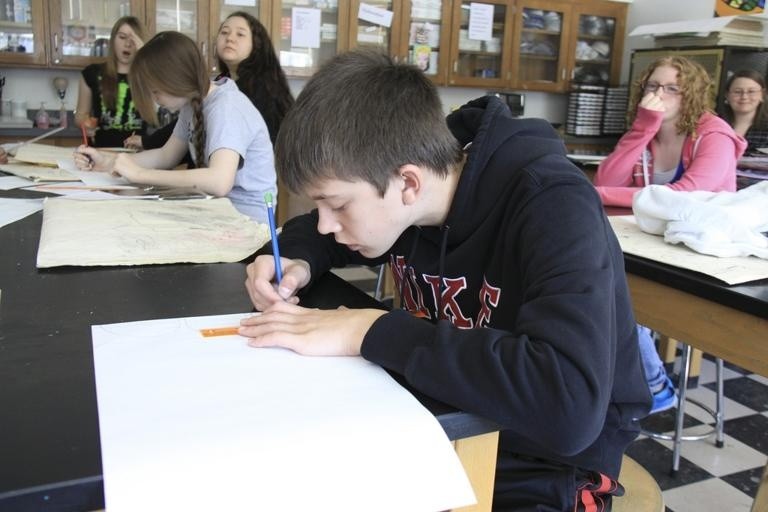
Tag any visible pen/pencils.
[5,127,64,153]
[80,121,92,163]
[127,130,136,149]
[264,191,283,286]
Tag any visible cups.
[80,108,100,134]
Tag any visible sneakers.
[647,378,675,414]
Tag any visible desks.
[0,148,499,510]
[568,153,768,193]
[603,207,768,512]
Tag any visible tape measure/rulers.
[200,327,240,337]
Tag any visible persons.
[214,11,295,145]
[73,15,159,147]
[236,48,653,511]
[75,30,278,230]
[717,68,768,156]
[589,54,748,422]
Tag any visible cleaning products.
[60,101,67,127]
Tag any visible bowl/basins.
[517,8,617,86]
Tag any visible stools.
[608,452,665,512]
[636,324,725,477]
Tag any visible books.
[0,138,144,190]
[628,14,768,48]
[566,78,629,137]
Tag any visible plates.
[564,80,630,139]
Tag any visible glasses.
[641,81,684,95]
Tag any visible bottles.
[34,101,53,132]
[0,98,13,121]
[12,98,28,119]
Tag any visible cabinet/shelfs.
[1,0,50,71]
[398,1,453,88]
[209,1,270,78]
[510,1,566,95]
[565,1,628,97]
[51,0,146,72]
[449,0,512,89]
[348,1,401,64]
[270,1,349,80]
[145,1,209,73]
